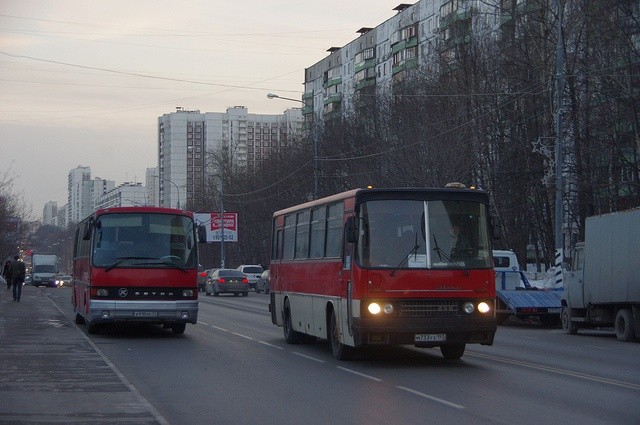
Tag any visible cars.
[24,260,32,284]
[197,264,208,291]
[205,268,248,296]
[55,272,72,287]
[255,270,270,294]
[206,268,218,277]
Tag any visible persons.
[3,260,13,289]
[441,222,473,261]
[8,256,26,302]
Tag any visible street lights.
[266,92,320,199]
[187,144,226,269]
[153,174,180,209]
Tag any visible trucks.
[559,206,640,342]
[31,252,58,288]
[492,249,563,327]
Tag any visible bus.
[71,206,207,335]
[268,182,497,361]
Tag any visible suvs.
[237,265,263,288]
